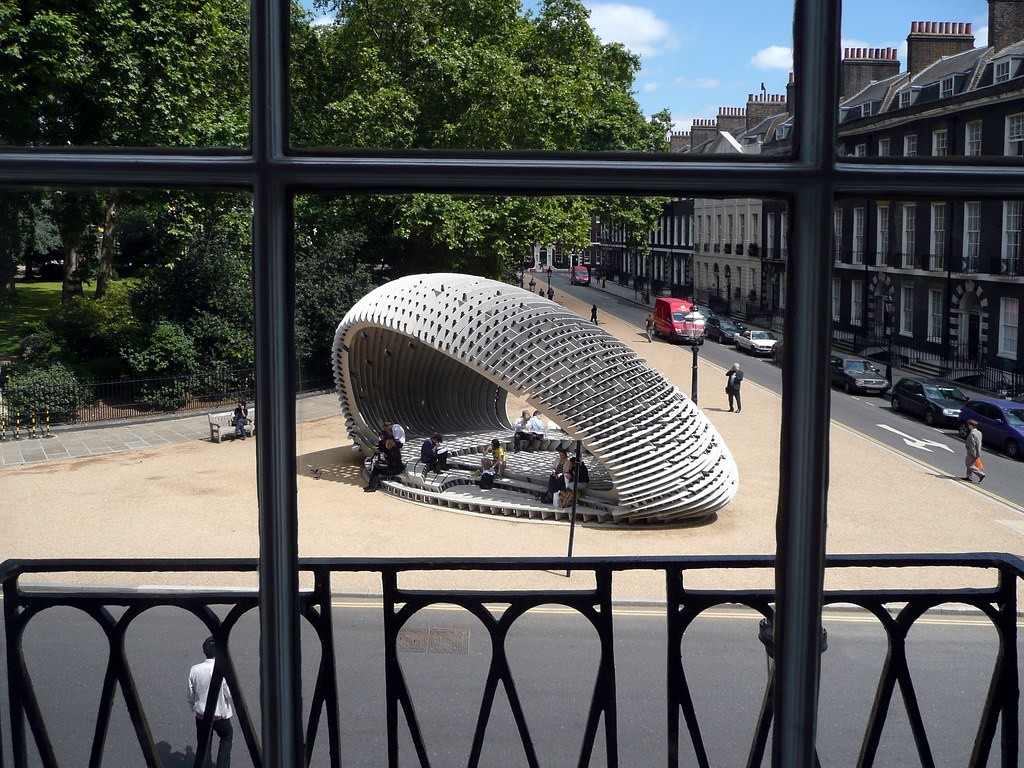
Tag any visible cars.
[958,398,1024,460]
[890,376,970,428]
[830,348,891,398]
[734,330,779,357]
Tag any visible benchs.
[208,407,255,444]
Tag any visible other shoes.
[526,448,533,453]
[728,407,734,412]
[433,469,443,474]
[576,491,581,498]
[961,477,973,481]
[231,434,235,440]
[240,435,247,440]
[500,476,502,480]
[979,475,986,483]
[363,486,375,492]
[441,466,449,471]
[735,409,741,413]
[514,448,521,454]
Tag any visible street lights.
[546,266,553,290]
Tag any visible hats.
[378,430,392,437]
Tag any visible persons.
[961,419,986,483]
[481,439,506,481]
[511,410,546,454]
[232,399,250,442]
[421,434,452,474]
[187,636,234,768]
[591,304,598,325]
[547,287,554,300]
[548,448,589,493]
[539,288,545,297]
[645,314,655,343]
[724,362,744,413]
[363,422,406,492]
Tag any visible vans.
[653,297,705,346]
[571,266,589,286]
[707,315,745,345]
[694,304,714,337]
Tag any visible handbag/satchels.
[726,387,729,394]
[480,469,495,491]
[975,457,984,470]
[231,414,239,426]
[541,487,573,508]
